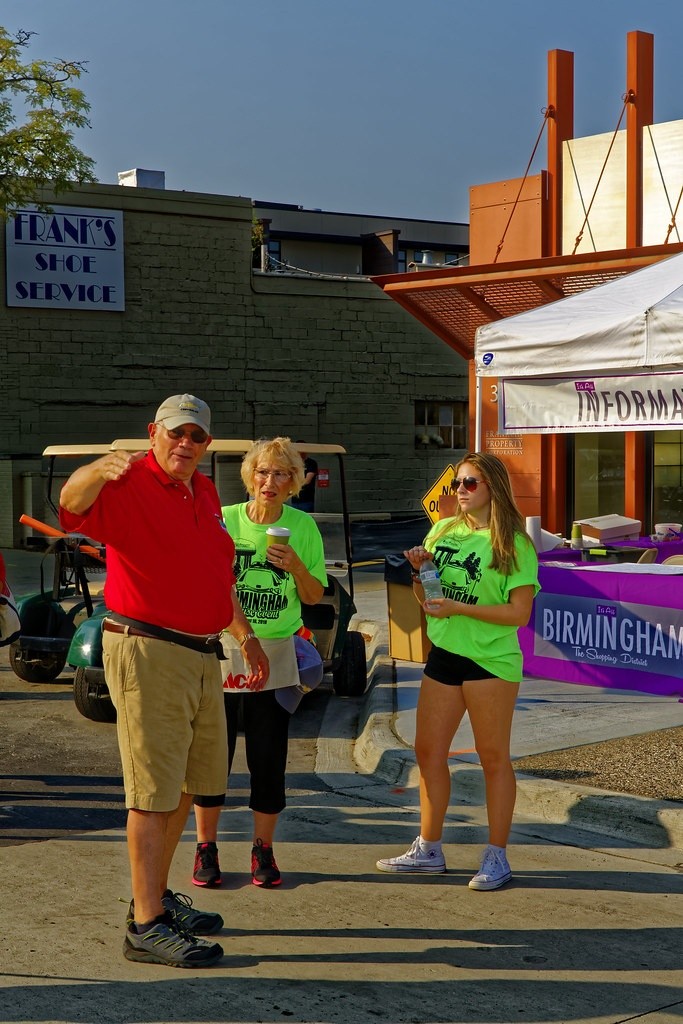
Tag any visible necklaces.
[474,525,490,530]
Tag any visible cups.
[267,525,290,559]
[571,524,583,548]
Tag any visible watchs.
[237,631,256,647]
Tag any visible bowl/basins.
[655,524,681,541]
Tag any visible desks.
[517,536,683,697]
[385,553,433,662]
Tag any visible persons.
[290,439,319,513]
[376,452,543,891]
[56,392,270,969]
[192,436,329,888]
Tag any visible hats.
[275,635,323,714]
[155,393,211,435]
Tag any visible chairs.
[211,451,249,506]
[636,548,658,563]
[661,555,683,565]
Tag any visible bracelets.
[410,566,420,575]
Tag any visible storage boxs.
[580,546,648,563]
[581,516,642,544]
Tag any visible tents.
[473,248,683,457]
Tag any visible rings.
[280,558,283,564]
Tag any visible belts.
[103,612,230,660]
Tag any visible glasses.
[168,427,208,443]
[451,477,487,492]
[256,468,292,483]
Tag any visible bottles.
[420,559,444,609]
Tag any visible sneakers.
[125,889,224,935]
[251,838,282,887]
[468,847,512,891]
[191,844,223,887]
[376,837,446,873]
[122,910,224,968]
